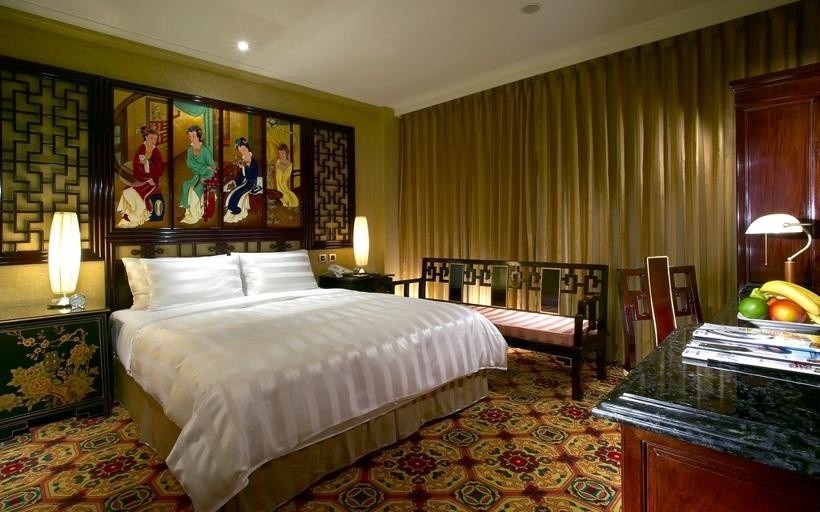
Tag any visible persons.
[178,123,218,225]
[223,136,259,224]
[115,123,167,228]
[272,143,300,208]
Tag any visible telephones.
[327,264,353,275]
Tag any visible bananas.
[749,280,820,325]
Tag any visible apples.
[768,300,807,323]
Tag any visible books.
[680,323,820,387]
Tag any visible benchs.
[389,257,609,401]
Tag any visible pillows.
[121,249,319,313]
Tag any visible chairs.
[615,255,704,376]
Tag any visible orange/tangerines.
[738,297,768,318]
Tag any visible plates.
[736,311,819,334]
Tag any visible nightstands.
[0,297,111,442]
[318,270,395,294]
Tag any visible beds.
[107,249,508,512]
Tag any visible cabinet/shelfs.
[589,323,820,512]
[728,62,820,297]
[704,295,820,335]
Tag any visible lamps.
[744,214,812,283]
[48,212,82,307]
[352,216,369,274]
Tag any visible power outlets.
[329,254,336,261]
[320,254,326,262]
